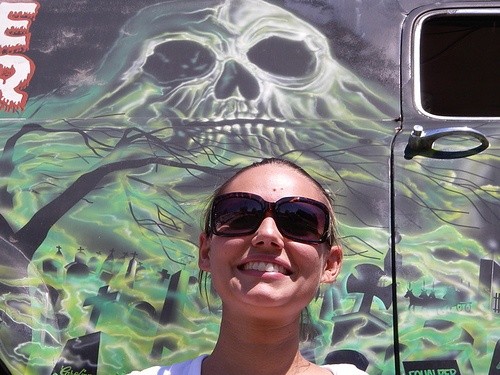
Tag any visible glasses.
[204,190,335,249]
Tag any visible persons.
[123,158,372,375]
